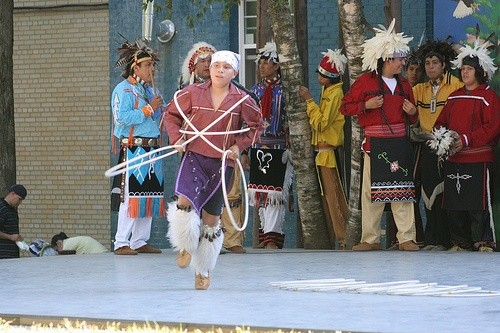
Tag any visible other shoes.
[448,246,466,252]
[478,245,494,252]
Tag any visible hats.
[5,184,27,199]
[208,50,241,73]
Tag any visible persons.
[387,36,435,250]
[177,41,250,255]
[0,184,27,259]
[410,35,467,251]
[298,47,352,252]
[338,17,423,253]
[161,49,265,290]
[51,231,110,255]
[104,31,169,257]
[429,21,500,253]
[244,40,290,251]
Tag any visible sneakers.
[397,240,419,250]
[137,244,162,253]
[176,249,191,269]
[264,241,279,250]
[351,242,381,251]
[195,271,210,289]
[227,246,246,254]
[115,246,138,255]
